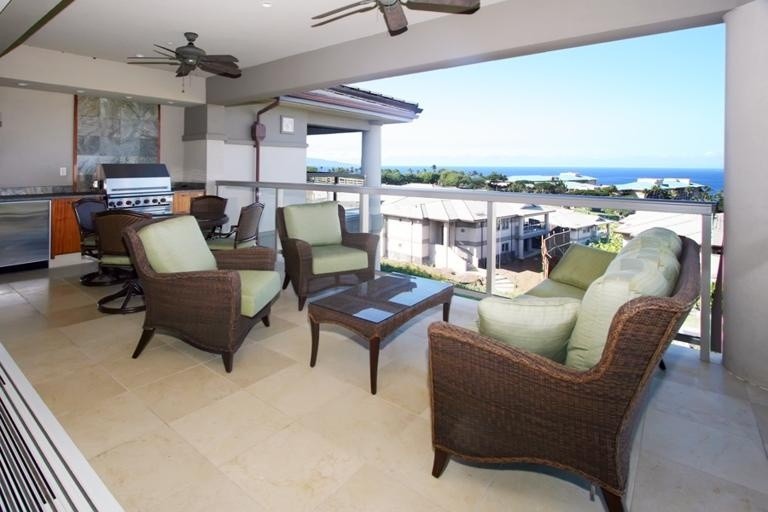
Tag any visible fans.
[307,0,484,37]
[123,31,241,84]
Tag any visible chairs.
[274,198,381,307]
[74,196,284,375]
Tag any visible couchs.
[430,227,702,511]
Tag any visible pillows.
[475,293,581,363]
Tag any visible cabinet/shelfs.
[49,197,105,260]
